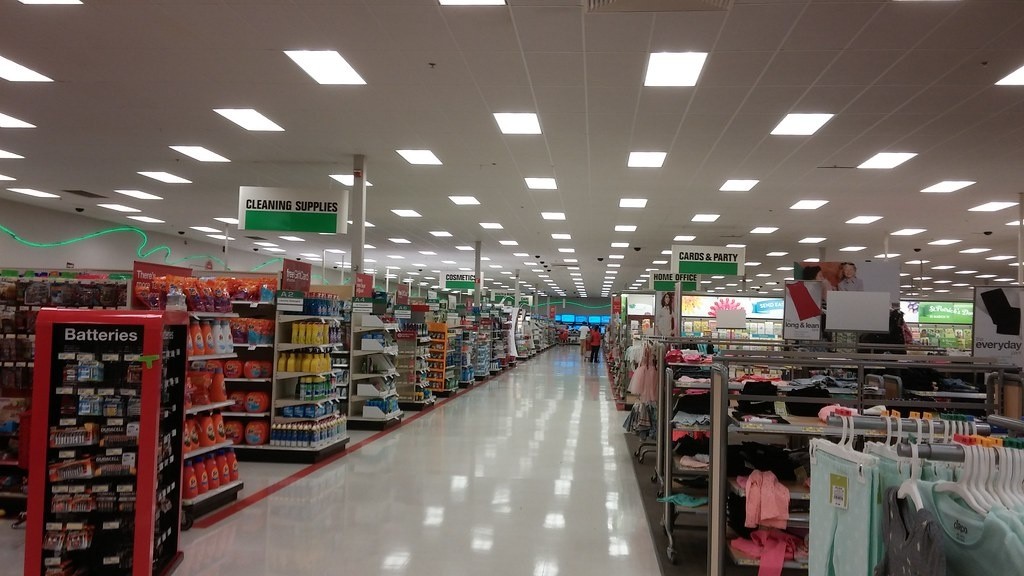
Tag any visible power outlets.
[968,285,1024,362]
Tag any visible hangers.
[810,414,1024,519]
[641,335,710,352]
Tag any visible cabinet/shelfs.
[707,356,1024,576]
[651,342,1004,498]
[664,361,1004,563]
[0,268,556,576]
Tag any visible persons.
[563,325,569,346]
[658,293,675,336]
[578,321,602,363]
[801,262,863,291]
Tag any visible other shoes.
[594,361,598,363]
[589,359,593,362]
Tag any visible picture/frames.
[783,280,822,341]
